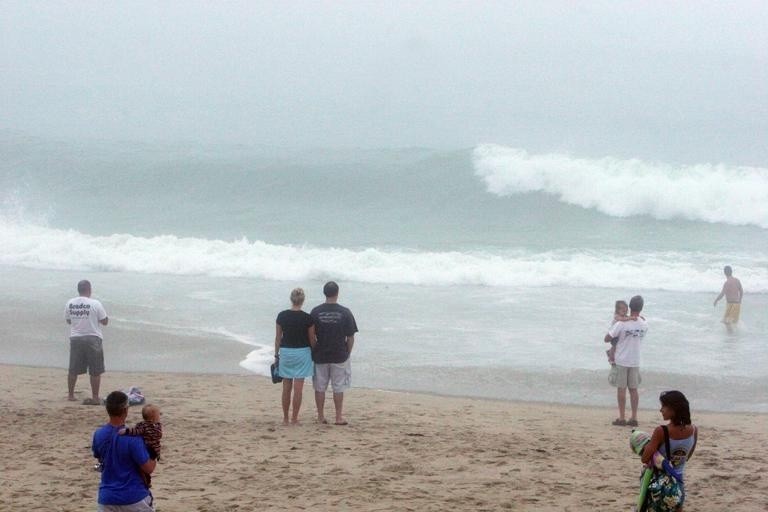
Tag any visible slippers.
[330,419,348,425]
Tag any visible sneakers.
[627,419,638,426]
[609,358,616,366]
[613,418,626,425]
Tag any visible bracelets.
[274,355,279,360]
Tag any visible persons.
[713,266,743,333]
[606,294,649,427]
[66,279,108,403]
[91,390,157,512]
[274,289,316,426]
[311,281,358,425]
[92,404,163,474]
[606,300,644,364]
[640,391,698,510]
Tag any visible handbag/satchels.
[645,463,685,512]
[270,362,283,383]
